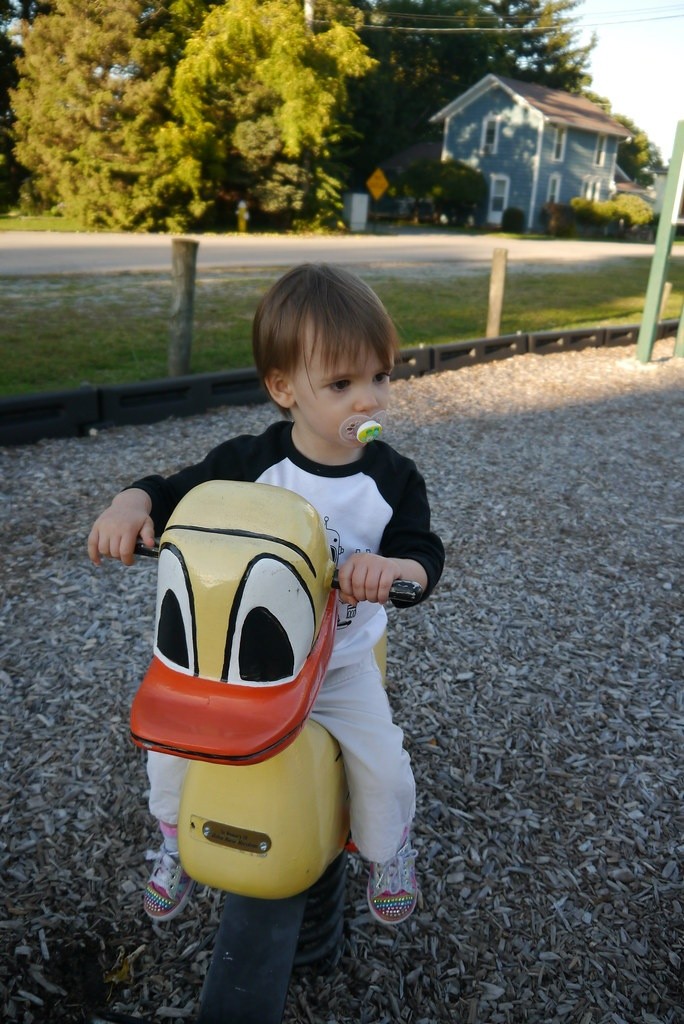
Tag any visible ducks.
[128,480,352,902]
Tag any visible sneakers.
[367,836,419,925]
[143,840,193,921]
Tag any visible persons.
[86,263,446,925]
